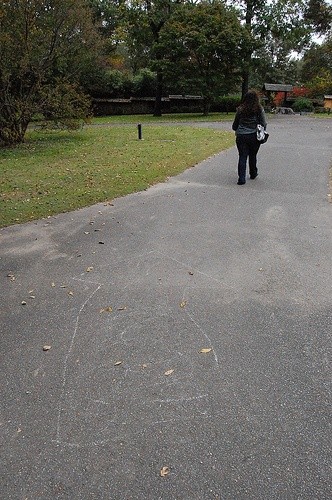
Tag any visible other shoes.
[237,181,246,185]
[251,174,257,179]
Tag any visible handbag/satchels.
[256,105,269,144]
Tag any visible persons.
[232,90,267,185]
